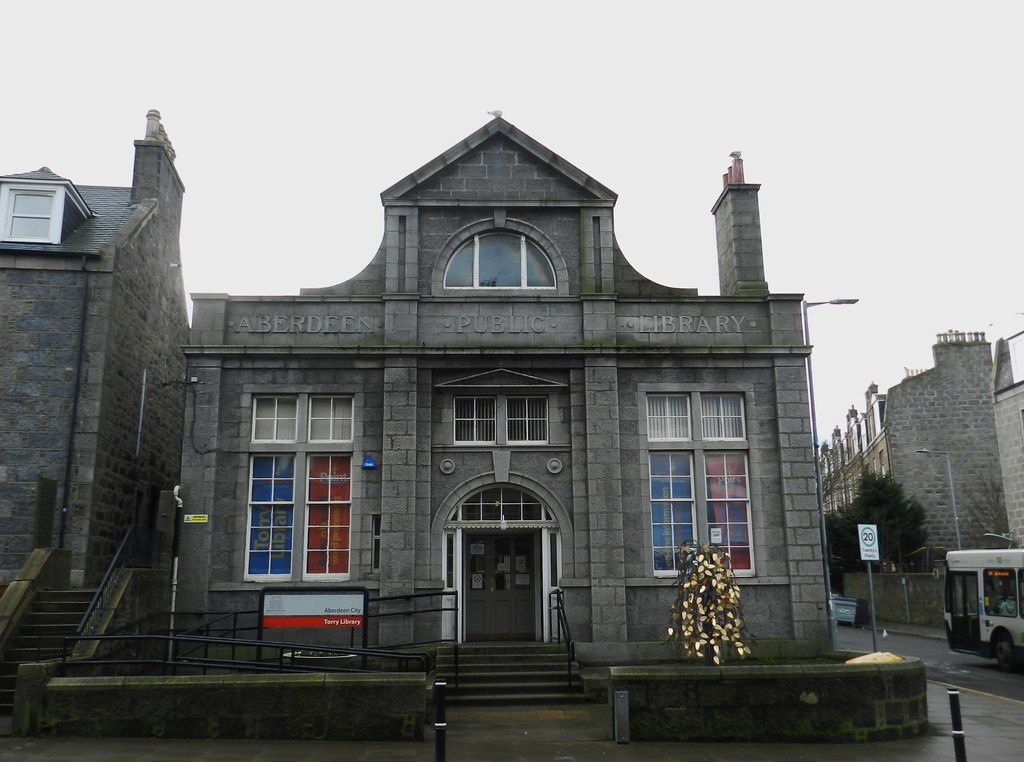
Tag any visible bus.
[934,550,1023,673]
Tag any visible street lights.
[802,298,859,649]
[915,449,962,549]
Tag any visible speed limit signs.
[858,524,879,560]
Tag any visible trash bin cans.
[830,596,866,628]
